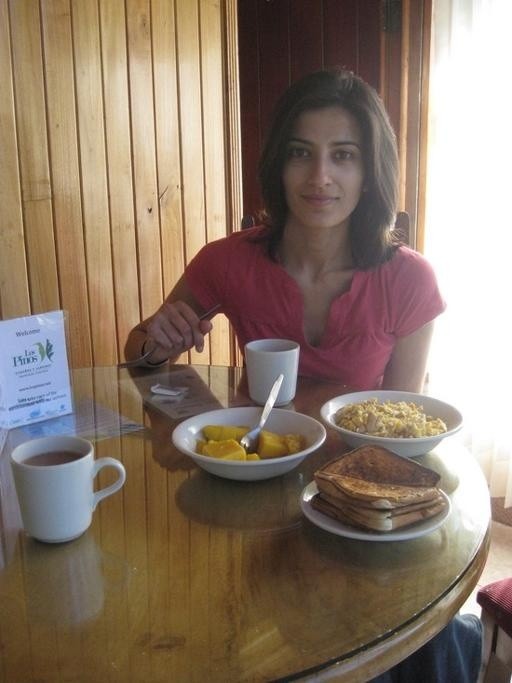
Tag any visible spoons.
[116,302,225,369]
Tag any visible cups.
[10,435,128,545]
[244,338,300,408]
[22,533,132,631]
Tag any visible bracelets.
[139,337,170,366]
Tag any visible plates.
[298,476,452,543]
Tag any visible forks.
[239,371,286,455]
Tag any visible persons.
[121,62,447,392]
[129,365,450,645]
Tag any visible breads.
[309,444,445,533]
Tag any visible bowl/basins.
[172,406,328,483]
[319,388,465,457]
